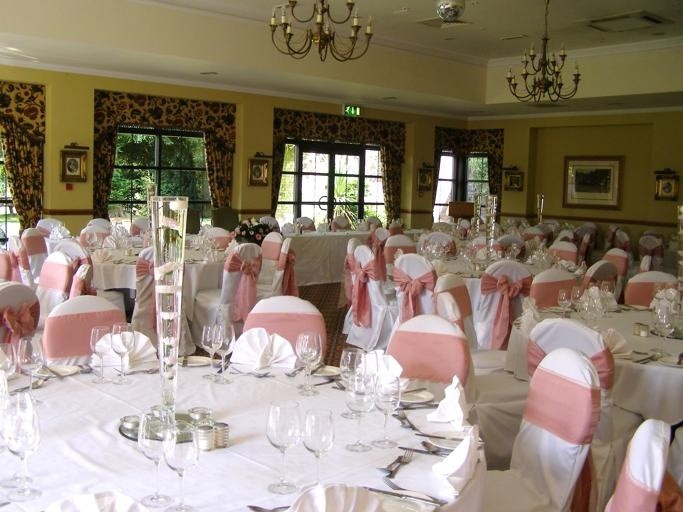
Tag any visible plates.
[311,365,340,376]
[657,356,683,367]
[37,364,78,377]
[428,430,485,450]
[178,355,211,367]
[402,388,434,403]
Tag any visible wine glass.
[295,329,321,395]
[0,393,45,504]
[557,282,623,327]
[111,321,135,384]
[165,422,199,512]
[646,283,680,361]
[90,325,113,386]
[16,335,47,407]
[372,374,406,449]
[345,374,375,454]
[202,323,224,380]
[138,407,175,508]
[212,323,236,385]
[341,348,368,421]
[0,344,17,406]
[264,401,302,497]
[304,412,338,488]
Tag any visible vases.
[484,194,498,261]
[537,191,545,225]
[471,191,481,238]
[149,195,190,427]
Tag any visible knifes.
[415,433,463,441]
[361,483,444,512]
[42,364,65,383]
[398,446,451,456]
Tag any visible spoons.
[376,454,405,474]
[245,500,291,512]
[420,441,484,462]
[391,413,413,429]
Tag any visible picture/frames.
[503,171,524,192]
[654,175,679,202]
[245,158,270,187]
[417,167,434,192]
[563,154,626,211]
[59,149,89,184]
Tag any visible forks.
[231,369,272,378]
[380,476,449,506]
[399,407,417,432]
[388,449,413,477]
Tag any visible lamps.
[269,0,375,63]
[502,0,583,106]
